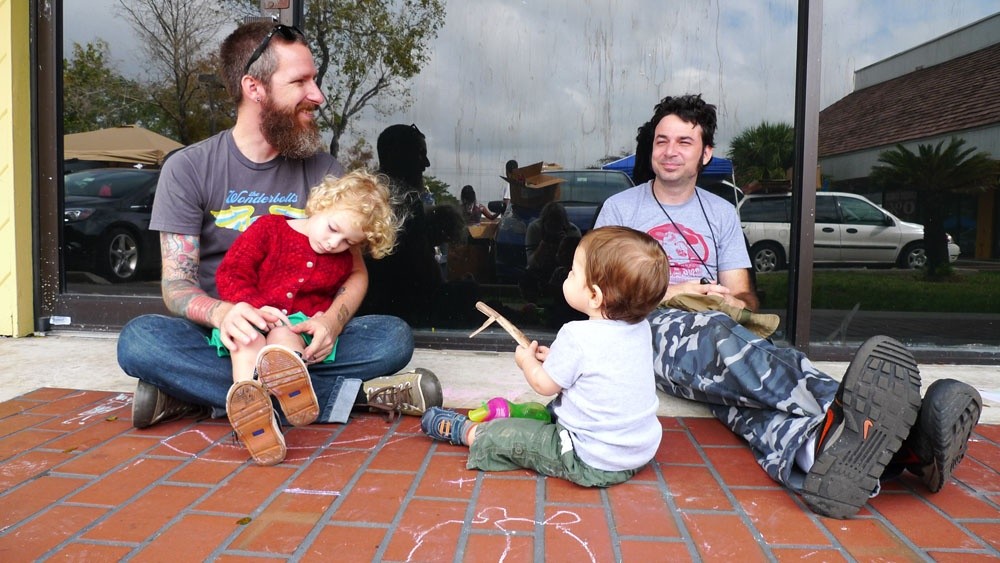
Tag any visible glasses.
[404,123,420,132]
[245,25,304,73]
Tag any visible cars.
[63,166,164,285]
[491,169,637,306]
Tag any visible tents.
[602,152,740,217]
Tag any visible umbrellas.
[64,124,186,166]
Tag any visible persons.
[364,124,446,287]
[119,22,444,429]
[594,94,983,519]
[421,225,670,489]
[215,167,399,467]
[524,202,583,284]
[458,184,500,225]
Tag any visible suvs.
[700,179,963,272]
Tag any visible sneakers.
[801,335,922,520]
[362,367,443,422]
[132,374,213,428]
[901,378,982,493]
[421,405,470,445]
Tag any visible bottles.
[423,185,435,205]
[468,397,552,425]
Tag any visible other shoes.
[256,346,320,426]
[225,379,289,467]
